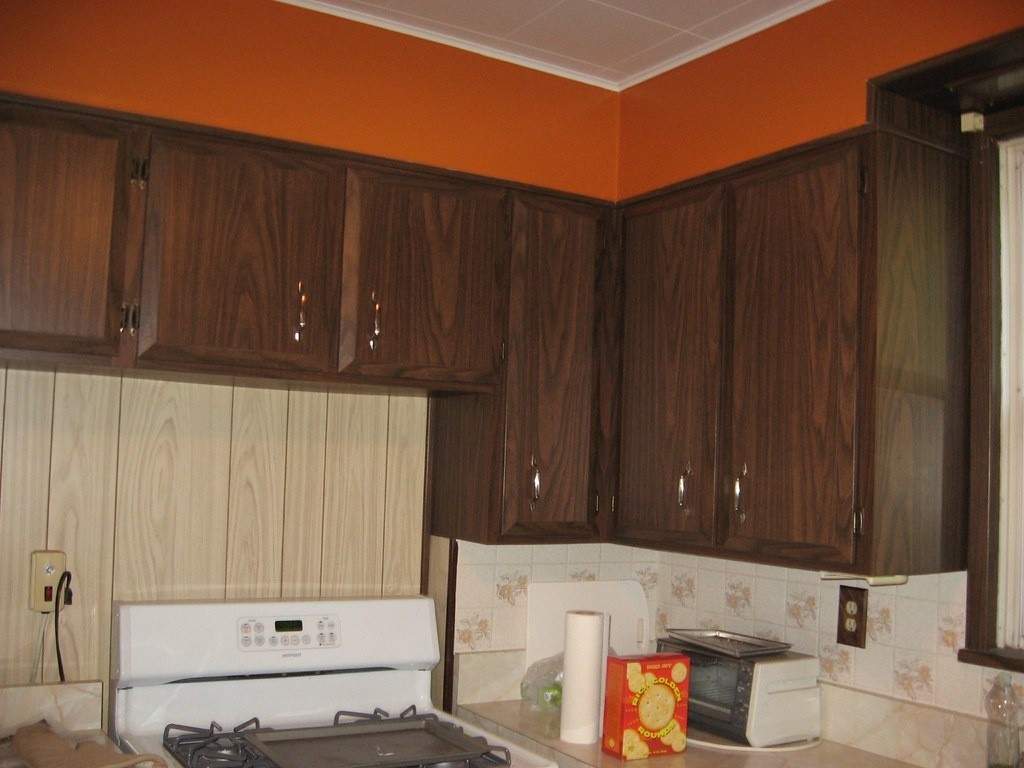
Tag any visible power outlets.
[837,585,869,650]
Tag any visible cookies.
[622,662,688,760]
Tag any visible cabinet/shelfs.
[0,90,967,578]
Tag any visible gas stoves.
[108,594,559,768]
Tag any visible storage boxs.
[602,653,690,762]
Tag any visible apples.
[538,682,562,710]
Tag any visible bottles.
[986,672,1020,768]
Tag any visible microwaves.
[656,638,820,748]
